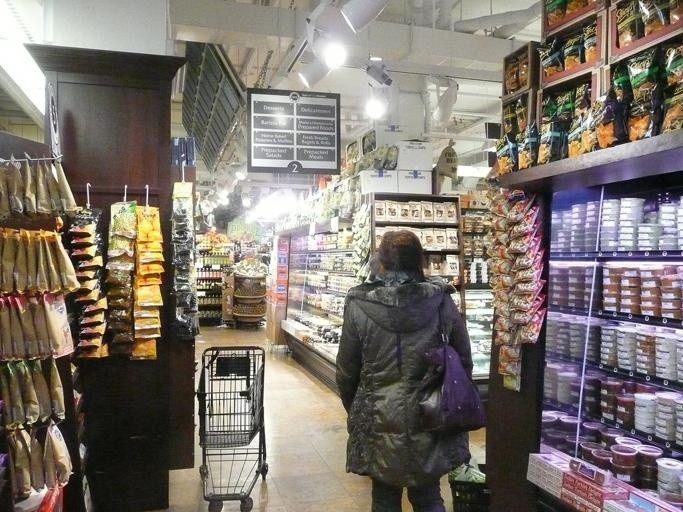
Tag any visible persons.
[332,228,476,511]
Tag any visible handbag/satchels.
[419,300,488,436]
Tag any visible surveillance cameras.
[367,64,393,86]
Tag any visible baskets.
[446,464,491,512]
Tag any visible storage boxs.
[499,0,682,140]
[327,120,433,201]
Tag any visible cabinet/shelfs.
[534,163,682,511]
[22,39,199,512]
[193,239,235,326]
[456,205,497,418]
[283,191,466,402]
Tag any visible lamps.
[428,74,459,124]
[337,0,388,33]
[296,53,332,89]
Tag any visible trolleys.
[192,341,274,512]
